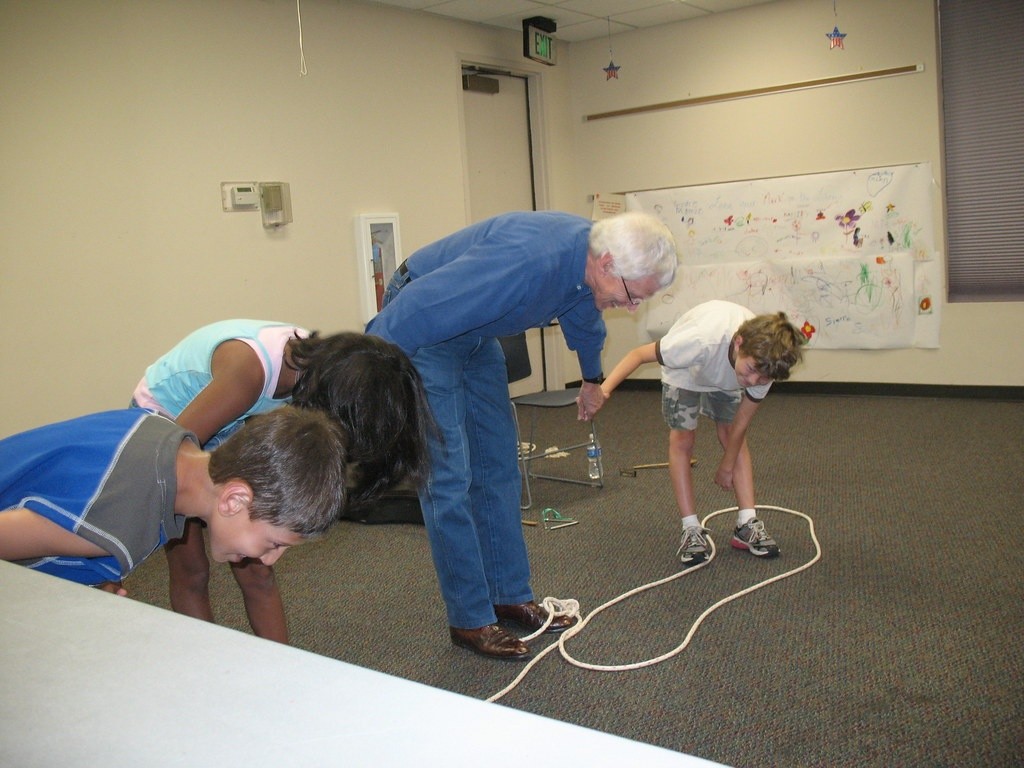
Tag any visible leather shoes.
[450,620,531,661]
[493,600,572,633]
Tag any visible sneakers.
[732,517,780,557]
[676,524,713,562]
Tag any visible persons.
[364,209,683,658]
[1,402,348,597]
[576,298,806,564]
[129,318,451,647]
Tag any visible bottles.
[587,433,603,479]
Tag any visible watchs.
[584,372,603,384]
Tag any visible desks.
[0,559,732,768]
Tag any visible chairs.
[495,331,605,510]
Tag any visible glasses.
[620,276,644,307]
